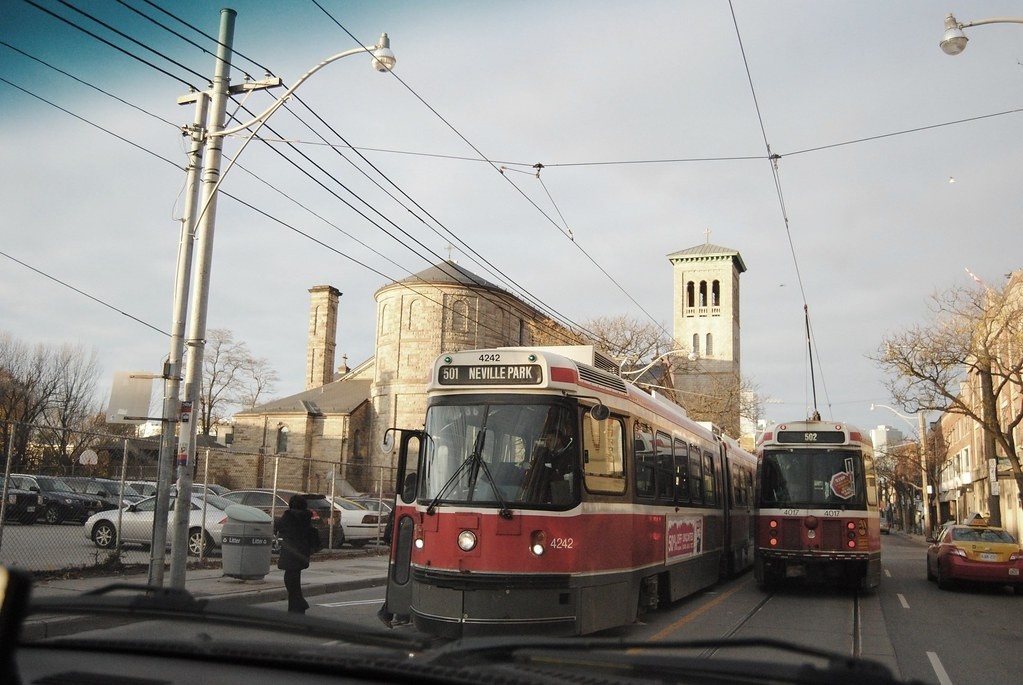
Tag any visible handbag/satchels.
[310,527,322,552]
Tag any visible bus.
[756,421,882,596]
[376,344,758,648]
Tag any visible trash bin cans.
[220,504,274,580]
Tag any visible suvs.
[223,486,346,552]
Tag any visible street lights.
[870,403,932,540]
[619,349,700,385]
[144,33,396,588]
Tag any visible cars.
[0,471,395,557]
[926,512,1023,597]
[879,518,891,535]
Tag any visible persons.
[275,495,319,615]
[529,430,580,505]
[375,470,419,629]
